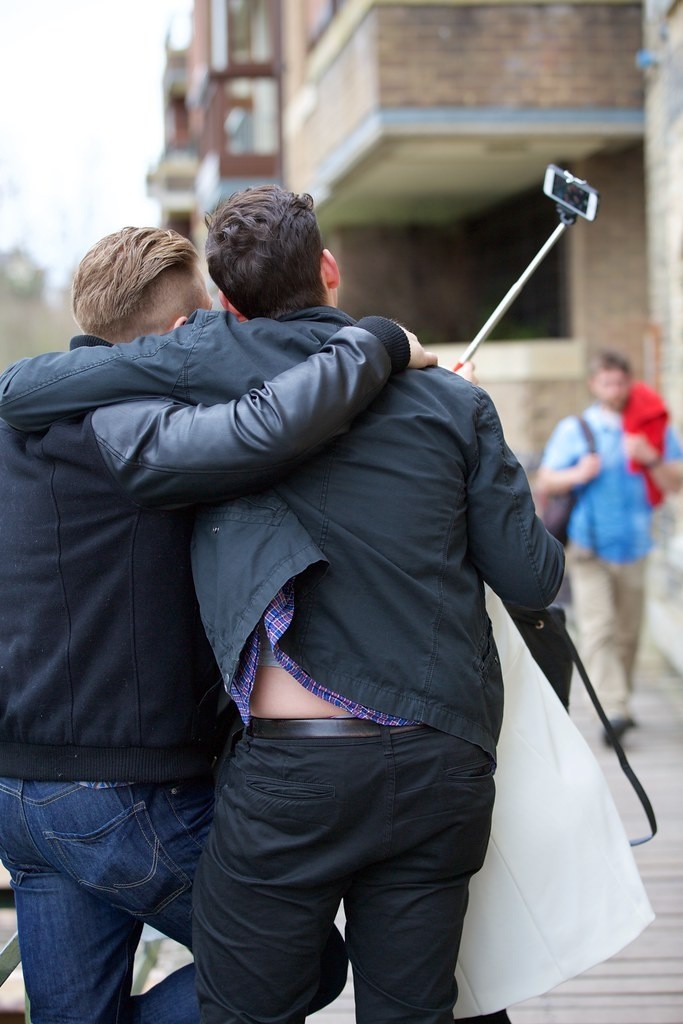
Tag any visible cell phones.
[543,164,600,222]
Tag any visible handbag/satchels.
[542,418,600,545]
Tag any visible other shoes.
[604,718,634,745]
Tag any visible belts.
[246,714,425,738]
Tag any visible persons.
[530,348,683,748]
[0,185,562,1024]
